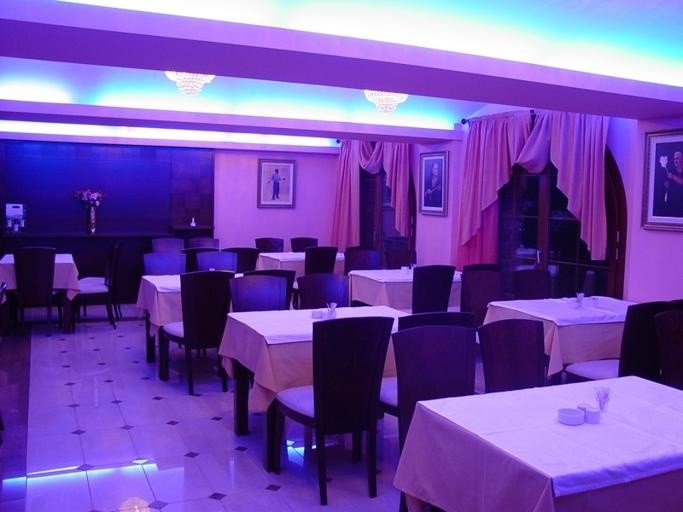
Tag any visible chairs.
[64,237,416,334]
[228,263,552,316]
[562,297,683,389]
[394,321,548,512]
[160,270,235,396]
[273,312,474,506]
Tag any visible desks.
[482,296,641,393]
[0,253,81,335]
[142,270,297,378]
[415,372,682,511]
[226,304,412,475]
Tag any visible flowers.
[74,189,105,207]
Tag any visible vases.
[85,207,97,232]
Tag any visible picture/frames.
[639,128,682,231]
[419,150,450,216]
[257,158,297,209]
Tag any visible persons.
[424,160,442,203]
[660,151,683,217]
[267,168,287,200]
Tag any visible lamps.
[364,89,408,112]
[164,70,216,95]
